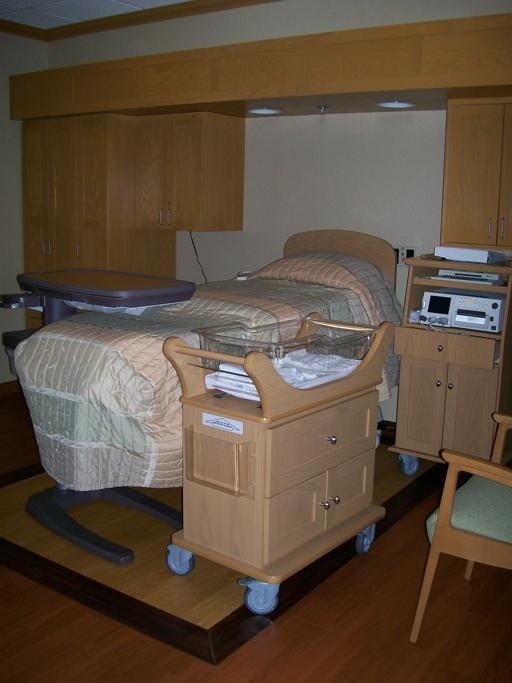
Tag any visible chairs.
[409,412,511,644]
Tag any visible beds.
[12,229,404,492]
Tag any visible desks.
[14,266,196,563]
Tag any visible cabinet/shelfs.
[129,110,244,231]
[439,98,512,251]
[387,252,512,475]
[23,112,176,330]
